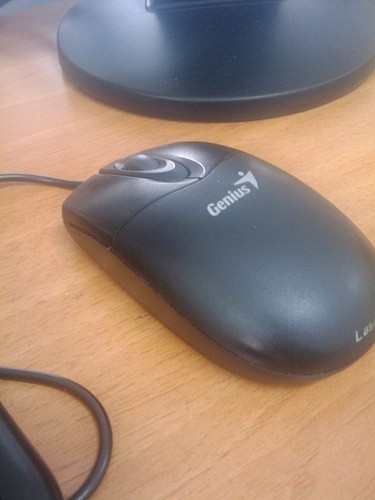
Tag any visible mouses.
[61,140,375,385]
